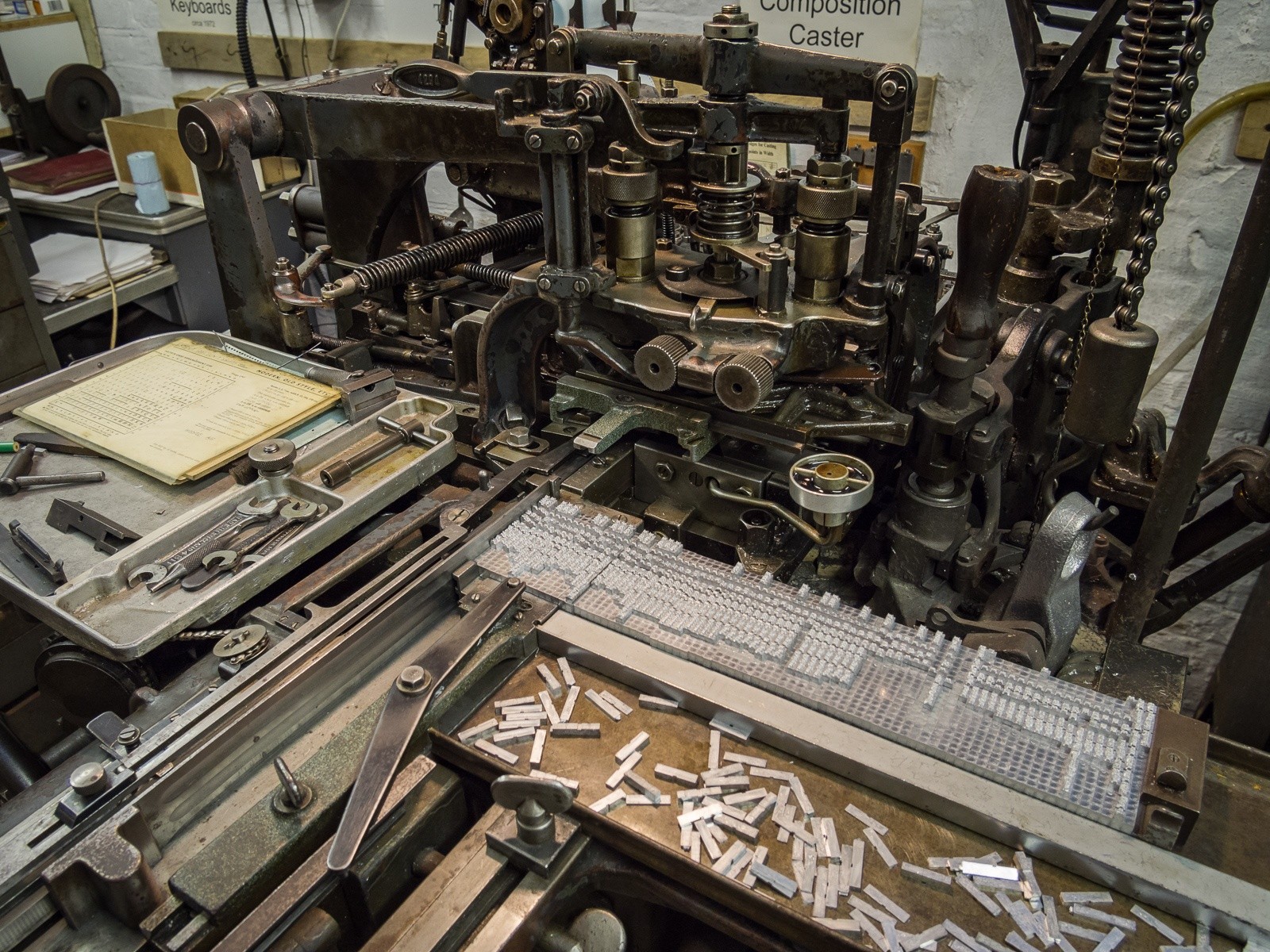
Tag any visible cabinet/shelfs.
[0,0,104,138]
[0,215,52,389]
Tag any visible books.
[4,148,117,195]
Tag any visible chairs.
[0,168,179,395]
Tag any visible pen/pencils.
[0,442,19,452]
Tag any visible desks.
[0,155,302,333]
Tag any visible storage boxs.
[100,108,266,210]
